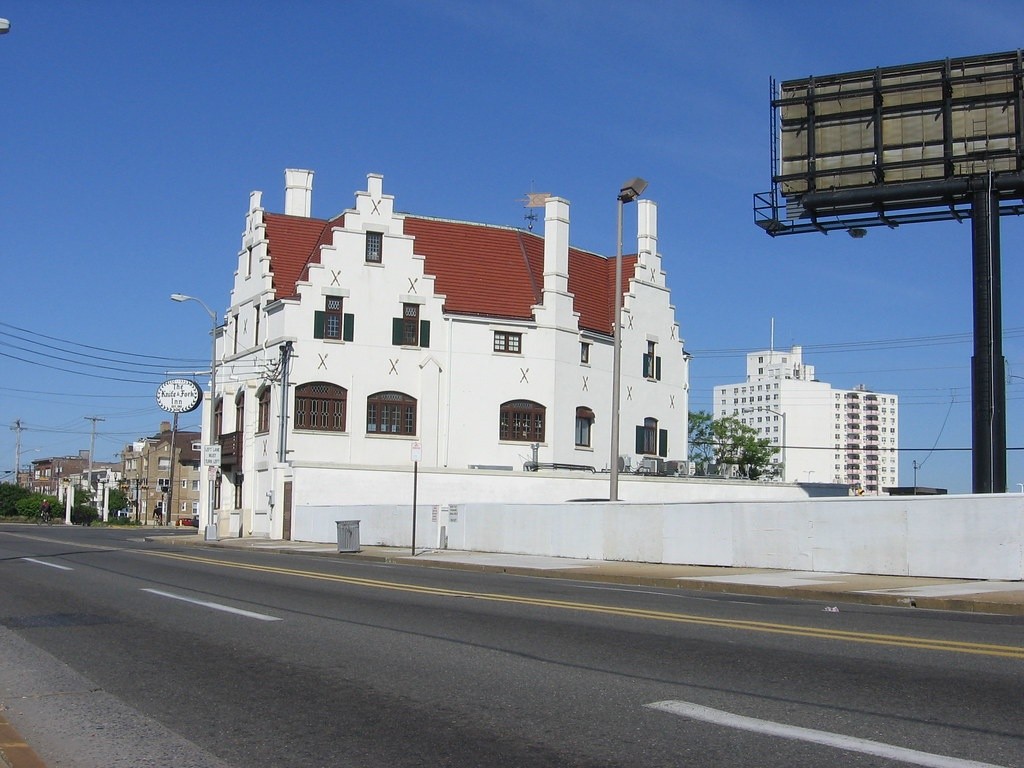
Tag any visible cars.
[176,517,193,527]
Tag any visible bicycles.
[35,509,55,528]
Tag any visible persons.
[40,499,49,523]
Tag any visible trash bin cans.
[335,519,361,552]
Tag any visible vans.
[192,514,200,527]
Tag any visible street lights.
[169,292,216,539]
[609,178,649,502]
[113,471,127,520]
[136,472,147,522]
[96,474,111,522]
[743,404,786,481]
[14,446,41,487]
